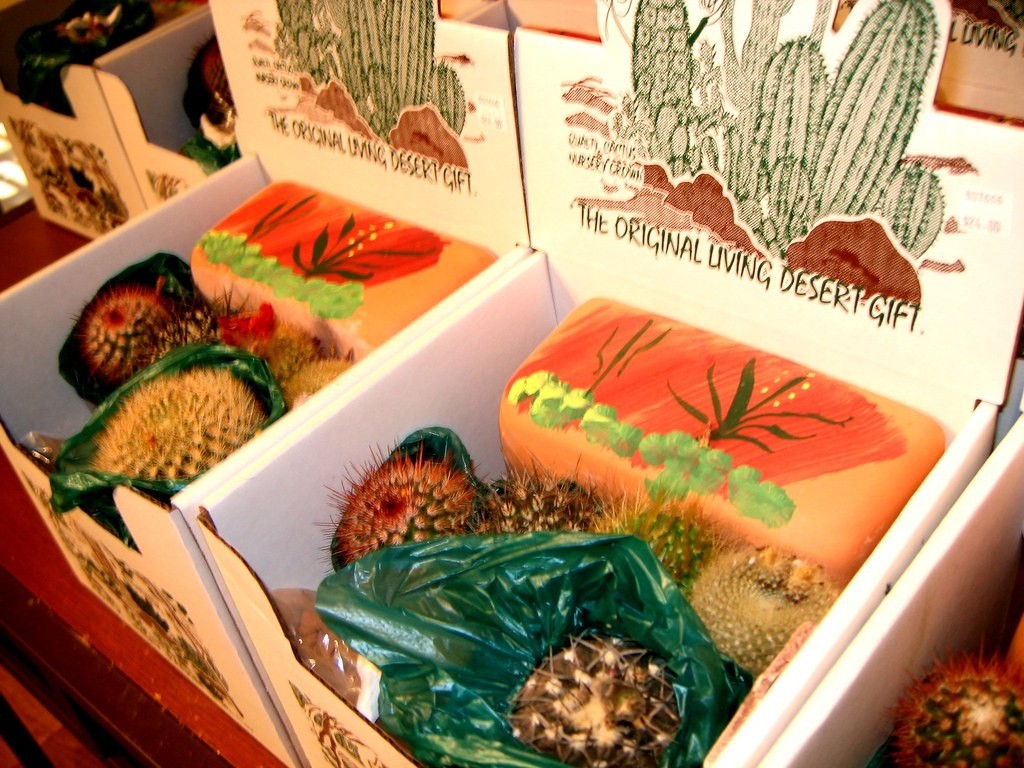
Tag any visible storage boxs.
[0,0,1024,768]
